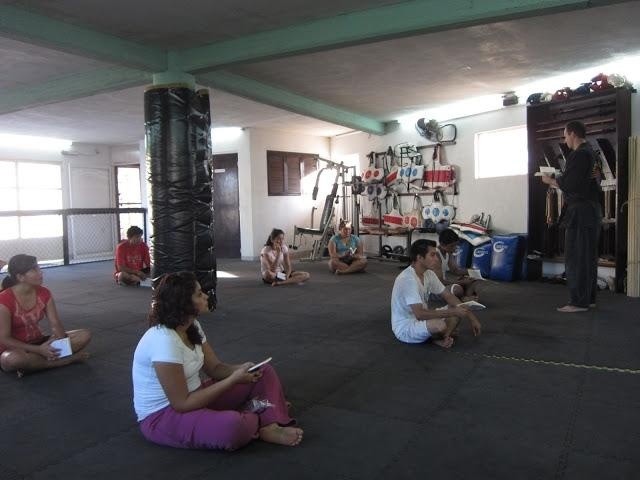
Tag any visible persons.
[328,218,368,274]
[542,121,602,313]
[390,239,481,349]
[431,229,486,302]
[113,226,150,286]
[131,272,304,451]
[260,228,310,287]
[0,254,92,378]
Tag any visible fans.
[415,115,457,143]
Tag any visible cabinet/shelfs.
[524,81,637,291]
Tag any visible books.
[463,269,487,281]
[140,278,152,287]
[535,165,563,179]
[277,272,286,281]
[49,337,73,358]
[349,247,358,255]
[457,300,486,311]
[247,356,273,372]
